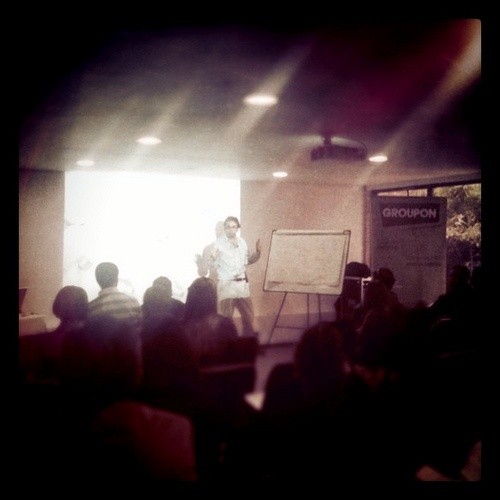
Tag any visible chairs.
[55,335,482,486]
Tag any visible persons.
[2,257,500,500]
[195,215,262,334]
